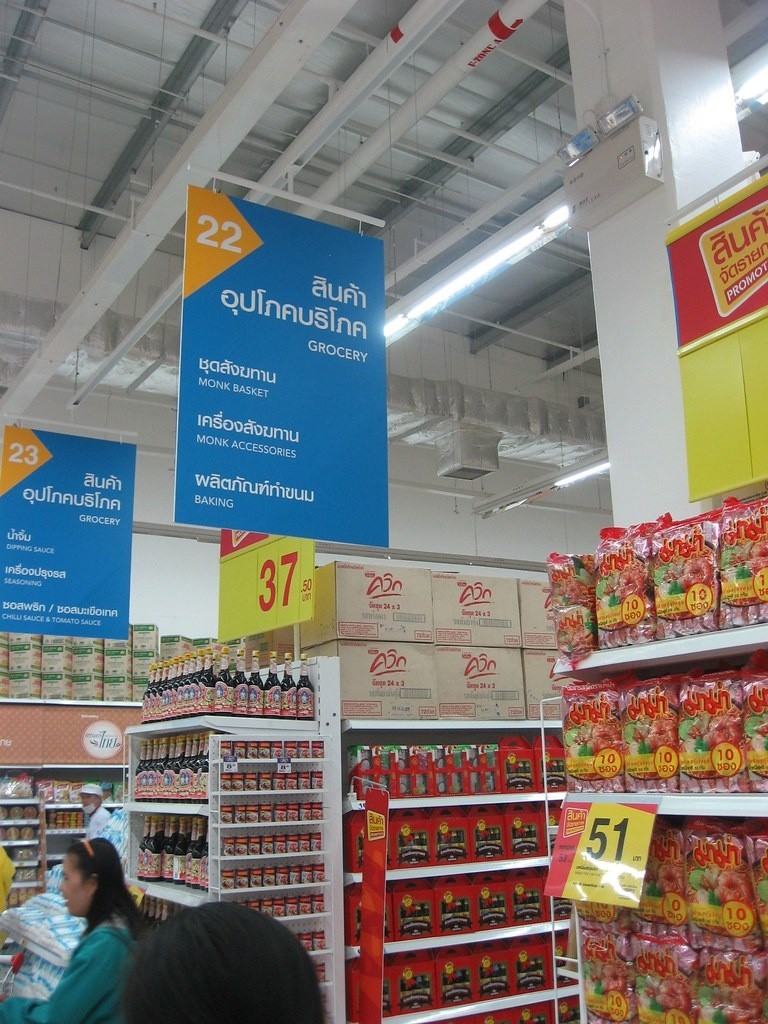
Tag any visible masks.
[82,800,98,814]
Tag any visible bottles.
[137,815,208,893]
[133,894,190,927]
[134,730,215,804]
[140,647,314,724]
[46,810,84,829]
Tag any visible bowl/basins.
[0,806,36,820]
[0,826,35,840]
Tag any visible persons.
[0,837,149,1024]
[126,902,324,1024]
[0,846,17,952]
[77,784,110,839]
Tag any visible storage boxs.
[244,560,562,722]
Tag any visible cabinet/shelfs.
[124,655,348,1024]
[0,764,131,946]
[539,621,768,1024]
[340,720,581,1024]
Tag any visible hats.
[79,784,103,797]
